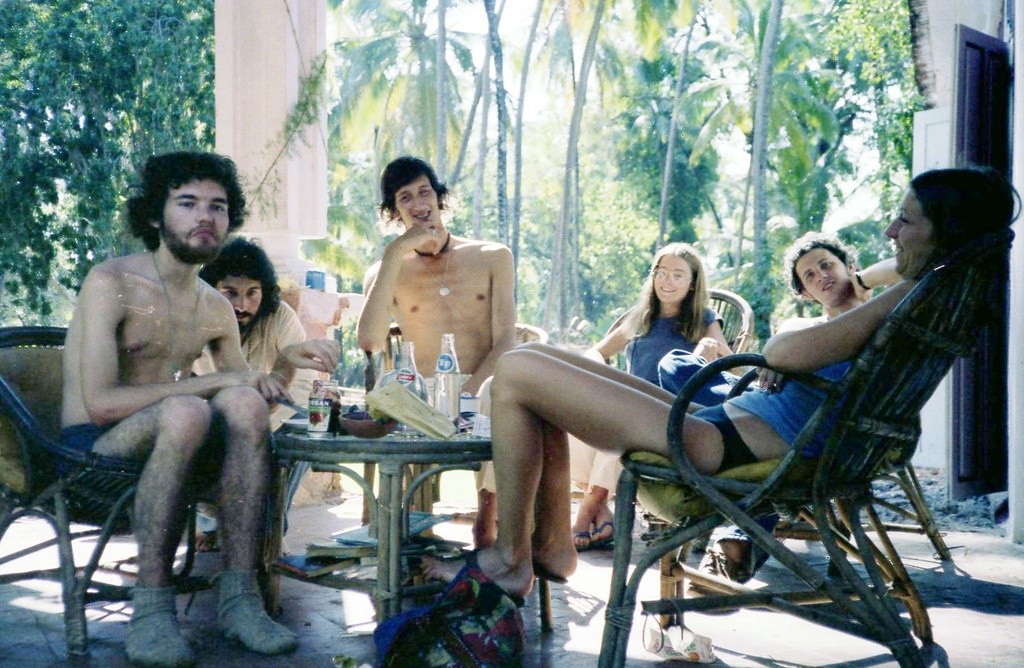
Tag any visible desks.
[269,423,555,638]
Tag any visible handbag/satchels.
[373,563,524,668]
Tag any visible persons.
[62,152,300,667]
[356,156,516,549]
[190,236,305,547]
[418,168,1021,607]
[719,230,904,583]
[571,242,734,552]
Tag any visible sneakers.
[689,539,769,596]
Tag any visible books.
[275,510,454,578]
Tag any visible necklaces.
[414,252,450,295]
[151,250,200,380]
[413,232,450,256]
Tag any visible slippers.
[532,555,568,583]
[432,550,527,608]
[591,522,615,545]
[571,531,591,550]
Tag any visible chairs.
[601,258,993,668]
[0,325,268,655]
[360,324,547,524]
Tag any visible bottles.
[307,379,340,432]
[395,341,422,440]
[432,332,462,437]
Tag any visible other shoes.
[125,587,194,668]
[217,570,297,655]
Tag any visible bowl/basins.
[339,412,398,438]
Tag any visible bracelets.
[856,272,871,291]
